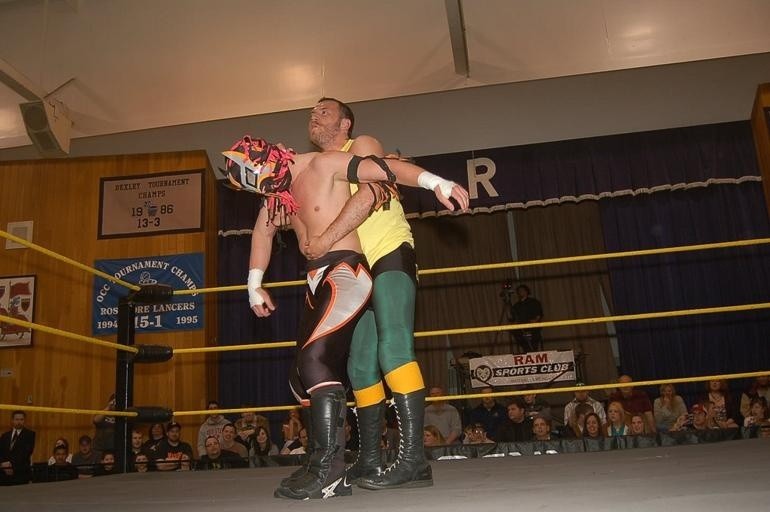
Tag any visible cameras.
[499,283,514,303]
[686,414,694,420]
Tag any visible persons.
[378,371,769,464]
[2,393,117,484]
[131,400,357,473]
[506,283,543,353]
[307,96,434,489]
[220,134,471,500]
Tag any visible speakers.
[18,100,72,157]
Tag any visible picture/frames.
[1,273,36,349]
[97,167,208,240]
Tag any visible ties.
[11,430,17,446]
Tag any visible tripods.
[488,301,535,355]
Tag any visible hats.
[167,422,181,433]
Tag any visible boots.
[273,384,433,500]
